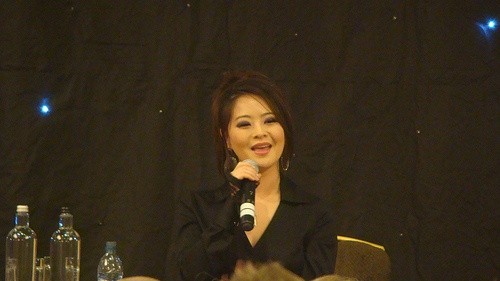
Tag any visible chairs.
[334,236,393,281]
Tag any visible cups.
[35,256,51,281]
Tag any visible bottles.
[5,205,37,281]
[97,242,124,281]
[50,207,81,281]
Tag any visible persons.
[158,67,338,281]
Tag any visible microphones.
[240,160,260,231]
